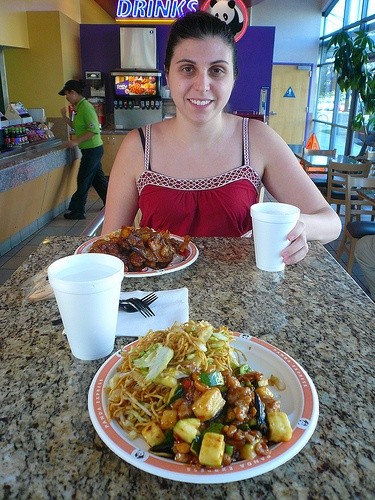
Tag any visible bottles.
[4,127,28,146]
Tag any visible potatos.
[83,225,190,271]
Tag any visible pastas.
[106,323,248,430]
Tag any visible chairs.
[303,147,375,274]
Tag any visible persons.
[354,235,375,303]
[58,80,109,220]
[100,9,342,265]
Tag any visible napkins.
[114,285,190,337]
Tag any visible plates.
[74,231,200,278]
[87,336,318,486]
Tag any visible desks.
[0,236,375,500]
[295,152,364,195]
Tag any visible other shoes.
[64,210,86,220]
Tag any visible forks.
[51,292,158,326]
[119,298,156,318]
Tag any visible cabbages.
[113,320,239,429]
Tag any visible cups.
[250,202,301,272]
[48,252,125,361]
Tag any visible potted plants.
[327,28,375,201]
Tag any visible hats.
[58,80,82,96]
